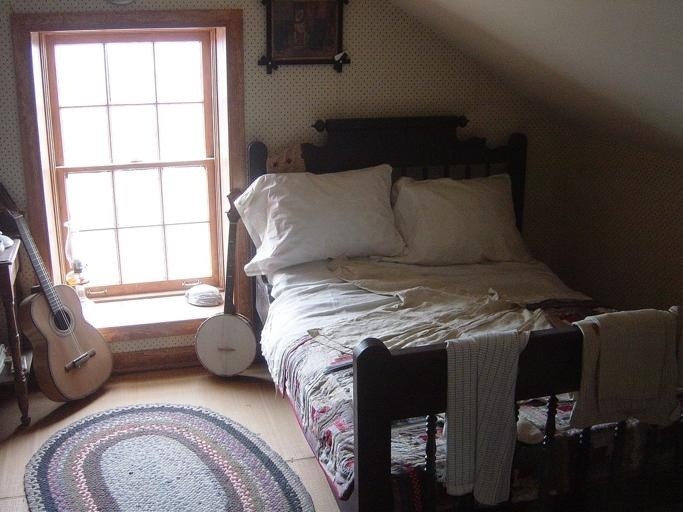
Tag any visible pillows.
[233,162,407,277]
[386,166,534,268]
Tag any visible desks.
[0,228,33,429]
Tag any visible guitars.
[1,180,112,401]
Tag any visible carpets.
[23,402,315,511]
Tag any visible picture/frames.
[255,0,353,75]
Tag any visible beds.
[243,110,682,511]
